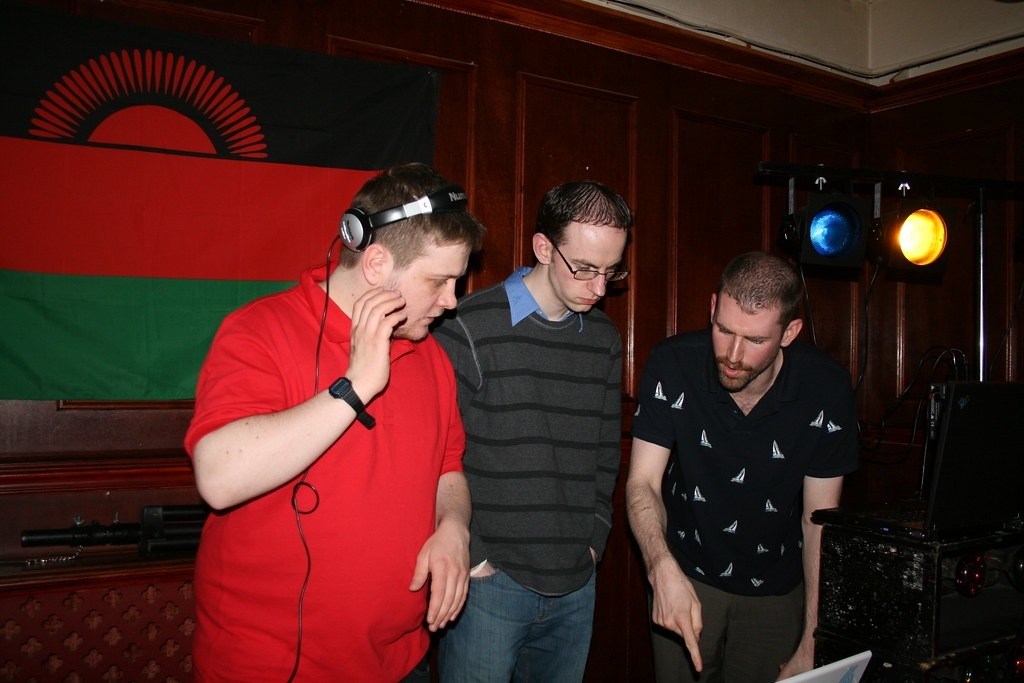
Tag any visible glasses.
[548,236,629,281]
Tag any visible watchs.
[328,377,376,427]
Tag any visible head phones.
[337,187,467,253]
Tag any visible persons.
[182,160,483,683]
[625,253,861,683]
[429,178,631,683]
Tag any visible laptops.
[812,380,1024,544]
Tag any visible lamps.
[778,170,950,286]
